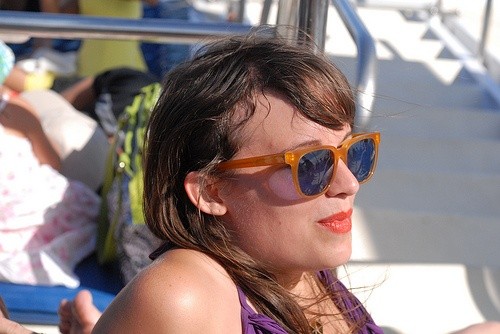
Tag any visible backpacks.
[96,83,165,293]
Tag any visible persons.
[0,0,163,334]
[87,23,500,334]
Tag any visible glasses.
[191,132,381,199]
